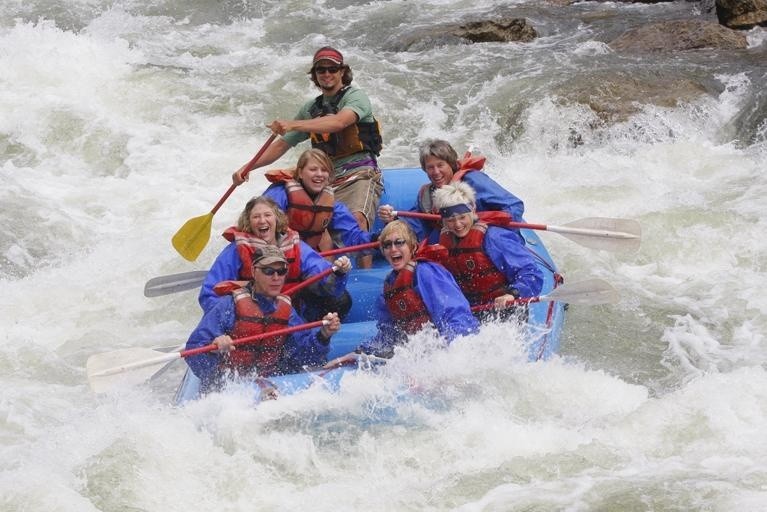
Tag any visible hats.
[313,48,343,66]
[252,246,288,266]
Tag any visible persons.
[184,244,340,399]
[231,46,384,269]
[198,195,353,325]
[377,138,525,242]
[411,181,544,326]
[323,218,481,373]
[260,148,382,264]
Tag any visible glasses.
[316,65,340,73]
[257,266,288,275]
[382,238,407,248]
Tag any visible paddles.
[472,278,620,321]
[171,131,277,262]
[144,241,378,298]
[391,210,641,254]
[324,352,388,367]
[87,320,330,394]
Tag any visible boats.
[172,165,563,443]
